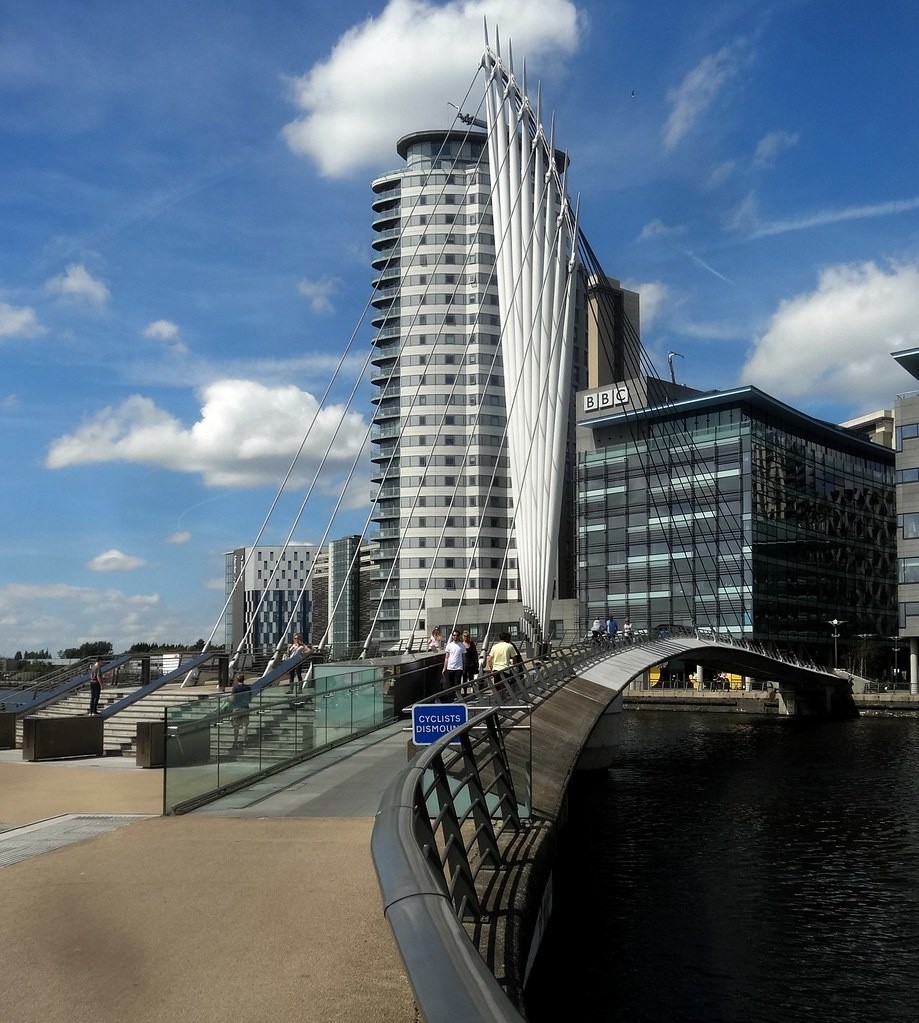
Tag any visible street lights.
[887,635,903,684]
[826,619,847,669]
[855,634,876,678]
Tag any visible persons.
[590,615,634,651]
[711,670,731,691]
[441,629,468,687]
[488,632,517,703]
[286,633,311,694]
[229,673,253,746]
[425,626,444,651]
[462,630,479,696]
[89,656,104,715]
